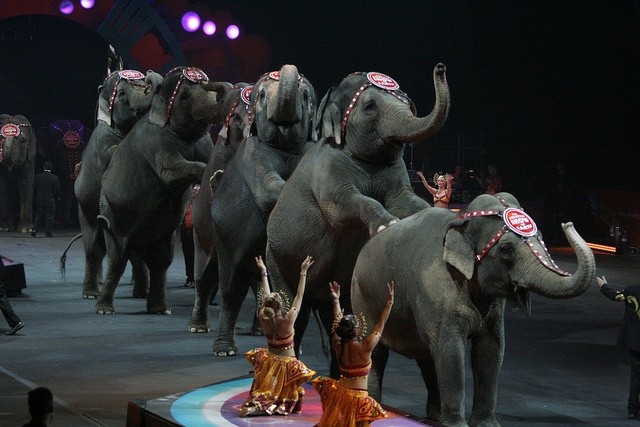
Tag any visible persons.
[415,169,455,209]
[309,279,396,427]
[58,169,77,223]
[20,385,55,427]
[29,159,62,238]
[238,253,315,419]
[594,272,639,421]
[0,258,25,336]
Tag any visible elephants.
[92,66,233,315]
[60,69,163,299]
[265,63,451,381]
[187,82,277,333]
[211,65,319,357]
[0,114,37,233]
[351,191,596,427]
[39,118,91,224]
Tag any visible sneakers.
[184,281,194,288]
[31,232,36,236]
[5,322,24,335]
[627,410,637,419]
[46,232,51,237]
[208,300,219,305]
